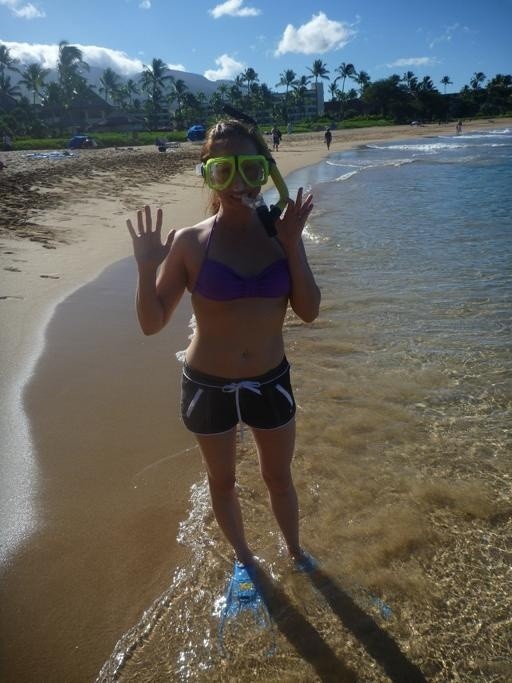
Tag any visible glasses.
[202,155,271,191]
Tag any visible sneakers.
[234,554,257,602]
[292,551,334,594]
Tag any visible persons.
[270,123,281,151]
[325,127,333,151]
[286,119,293,135]
[456,120,464,135]
[124,115,330,602]
